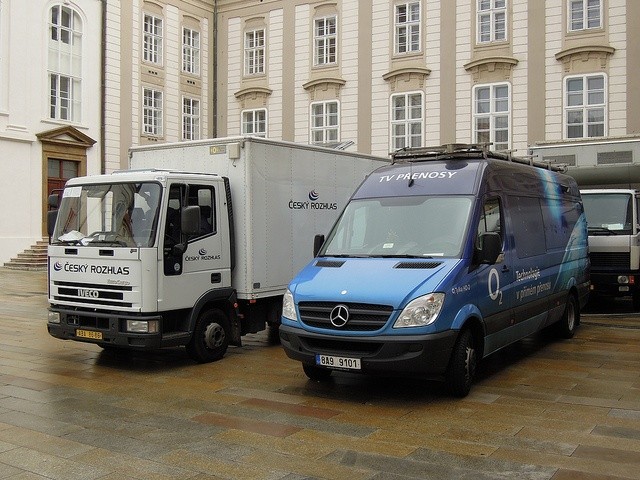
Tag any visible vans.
[278,143,592,397]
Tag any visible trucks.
[578,189,640,302]
[47,136,391,362]
